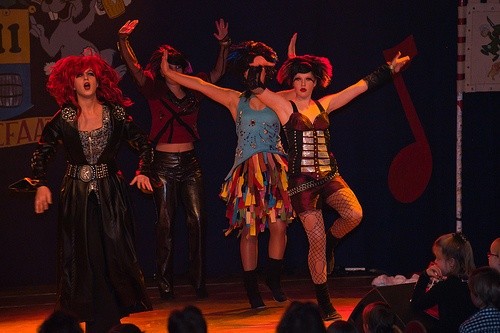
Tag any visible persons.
[487,238,500,273]
[413,232,478,333]
[459,267,500,333]
[36,303,208,333]
[246,52,410,321]
[31,55,154,333]
[160,33,297,308]
[275,298,428,333]
[118,19,231,301]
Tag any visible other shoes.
[196,287,208,297]
[157,280,176,299]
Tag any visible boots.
[326,228,341,275]
[314,283,341,319]
[265,259,286,302]
[243,269,265,309]
